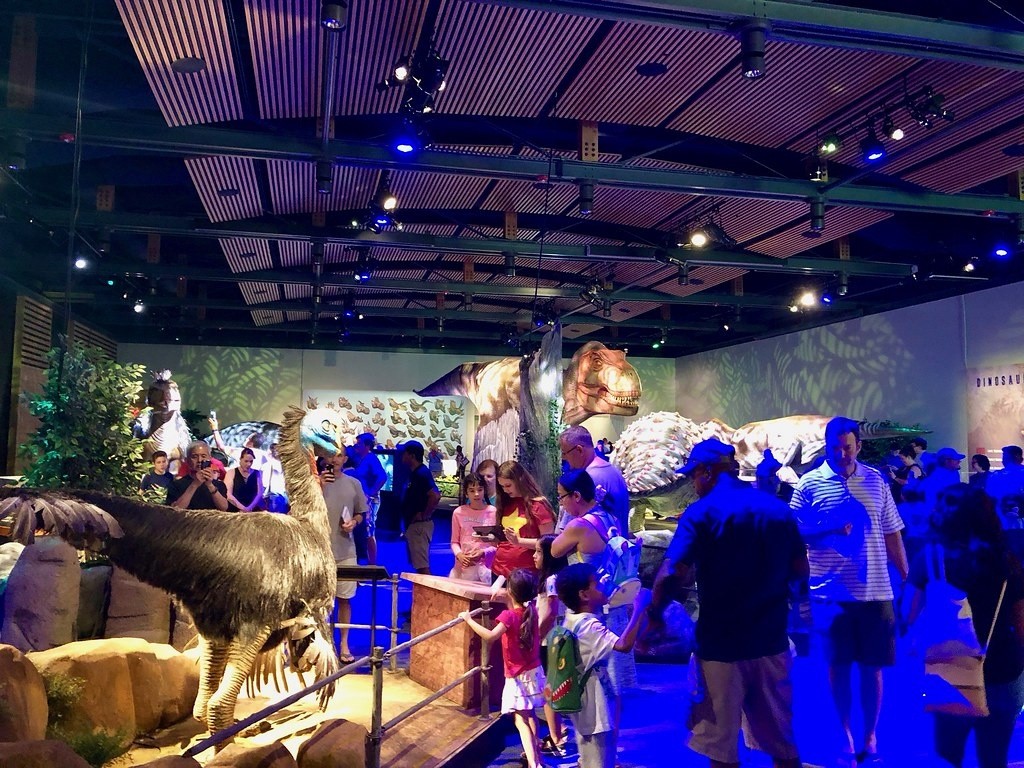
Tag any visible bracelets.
[212,429,219,432]
[353,518,359,526]
[210,487,218,494]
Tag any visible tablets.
[474,526,507,542]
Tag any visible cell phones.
[325,465,334,482]
[201,459,211,470]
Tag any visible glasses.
[562,443,585,457]
[557,489,582,502]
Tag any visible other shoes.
[537,736,567,755]
[521,751,528,760]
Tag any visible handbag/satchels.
[921,654,989,716]
[896,543,980,654]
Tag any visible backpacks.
[576,512,642,611]
[544,617,608,713]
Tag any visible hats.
[396,441,424,456]
[938,447,966,461]
[675,438,735,474]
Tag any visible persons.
[882,437,1024,768]
[532,533,569,678]
[394,440,441,623]
[551,424,650,768]
[428,445,465,477]
[223,449,263,514]
[342,432,387,582]
[647,438,809,768]
[262,442,290,516]
[166,440,228,512]
[138,451,175,504]
[489,460,556,588]
[448,460,502,583]
[790,416,912,768]
[208,418,264,471]
[317,443,370,662]
[458,568,547,768]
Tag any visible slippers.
[339,650,355,665]
